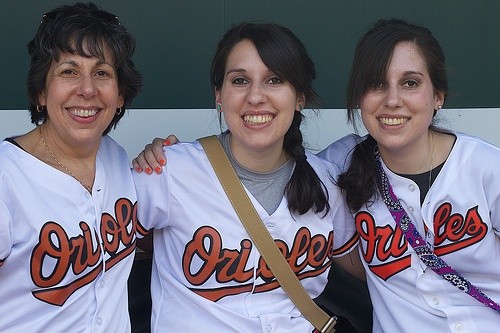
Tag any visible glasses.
[40,6,121,27]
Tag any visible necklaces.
[428,125,434,192]
[39,127,91,190]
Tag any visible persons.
[131,20,368,333]
[0,0,137,333]
[131,18,500,333]
